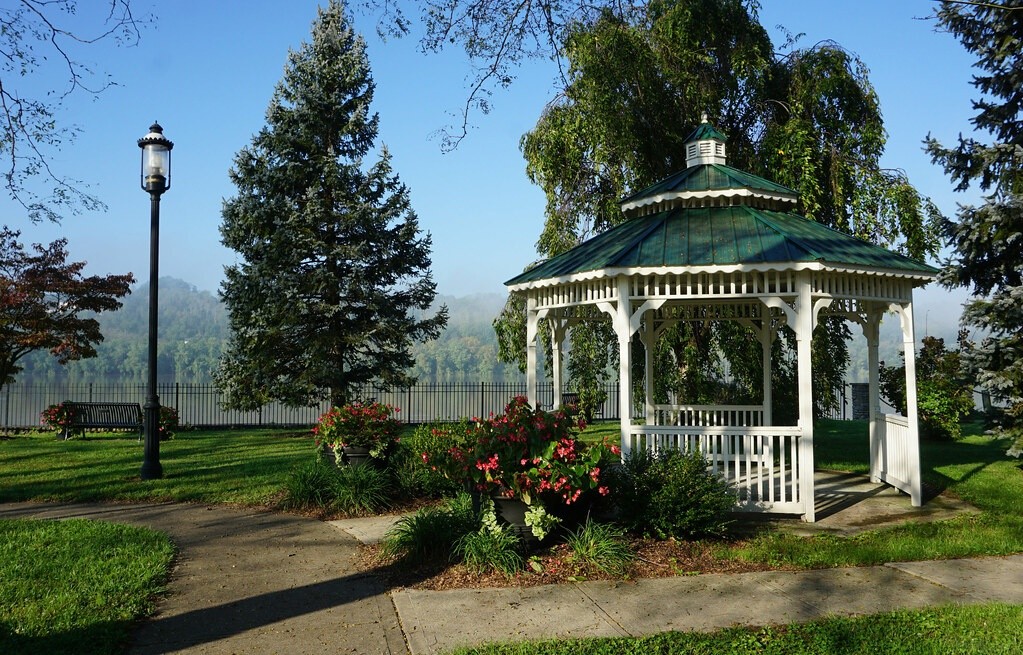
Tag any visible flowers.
[422,396,621,541]
[310,401,403,471]
[38,401,83,436]
[141,405,181,435]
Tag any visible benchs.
[63,400,143,442]
[537,392,607,424]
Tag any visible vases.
[55,427,71,440]
[469,488,570,554]
[323,445,383,468]
[158,430,169,441]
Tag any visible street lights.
[136,120,174,482]
